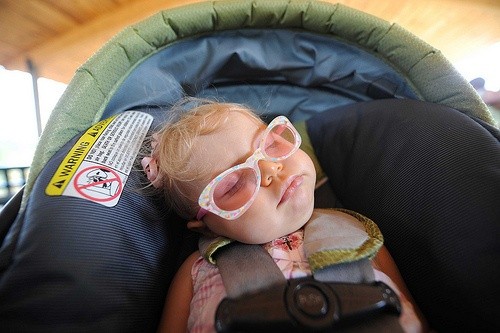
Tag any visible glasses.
[196,114,302,221]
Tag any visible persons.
[140,97,437,333]
